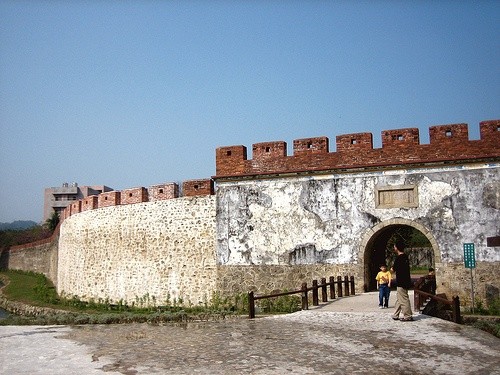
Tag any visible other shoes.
[379,304,388,308]
[392,314,413,321]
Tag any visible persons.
[427,266,435,276]
[375,264,393,309]
[390,241,412,322]
